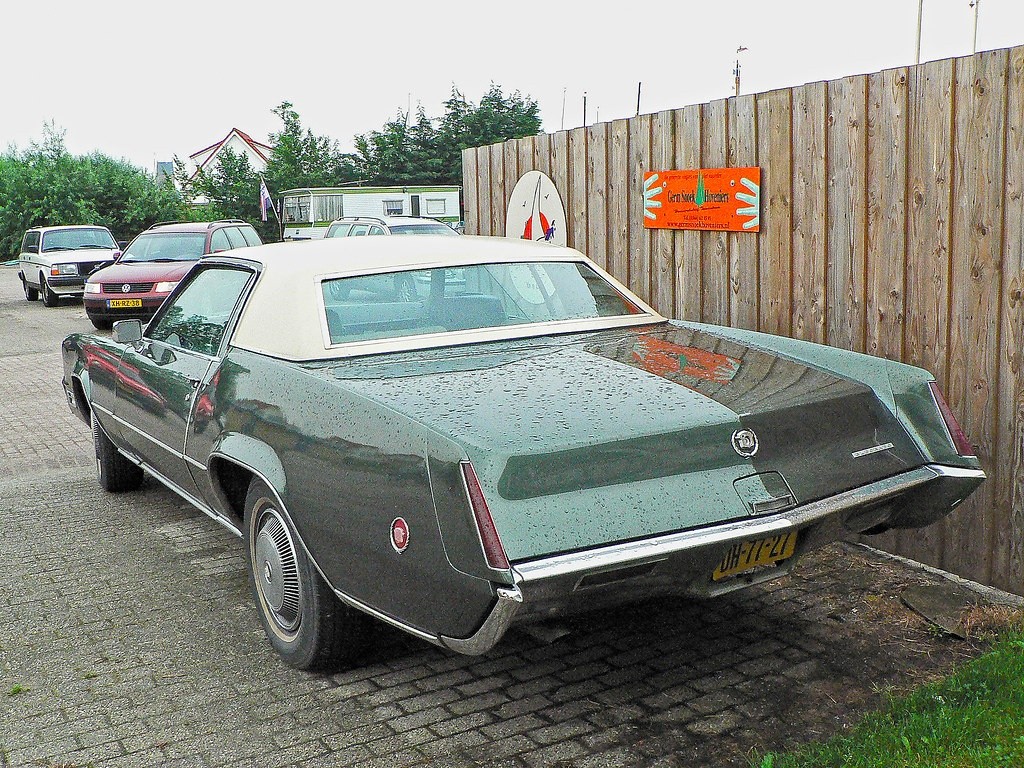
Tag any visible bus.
[277,186,462,241]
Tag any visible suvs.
[84,218,262,330]
[324,215,461,237]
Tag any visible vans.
[19,226,128,308]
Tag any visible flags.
[260,177,272,222]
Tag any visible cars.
[60,236,986,671]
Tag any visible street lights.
[969,0,979,54]
[735,45,748,95]
